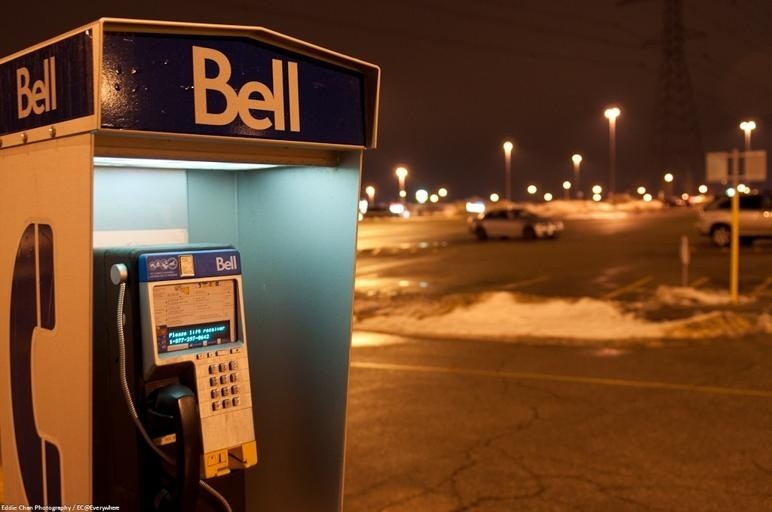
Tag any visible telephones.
[93,243,257,512]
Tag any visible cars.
[359,202,406,219]
[466,204,569,243]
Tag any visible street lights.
[603,106,621,206]
[739,119,757,153]
[361,138,604,211]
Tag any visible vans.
[694,189,772,250]
